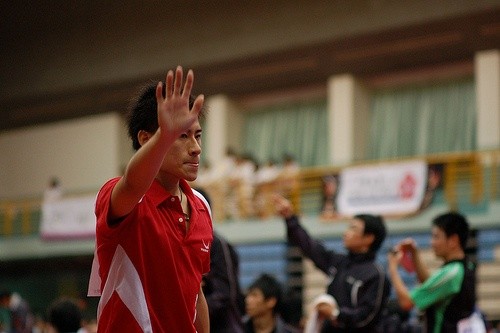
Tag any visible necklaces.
[179,184,190,223]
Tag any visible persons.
[270,193,393,332]
[241,272,304,333]
[94,66,214,333]
[0,293,90,333]
[387,211,477,333]
[43,177,63,204]
[193,146,297,200]
